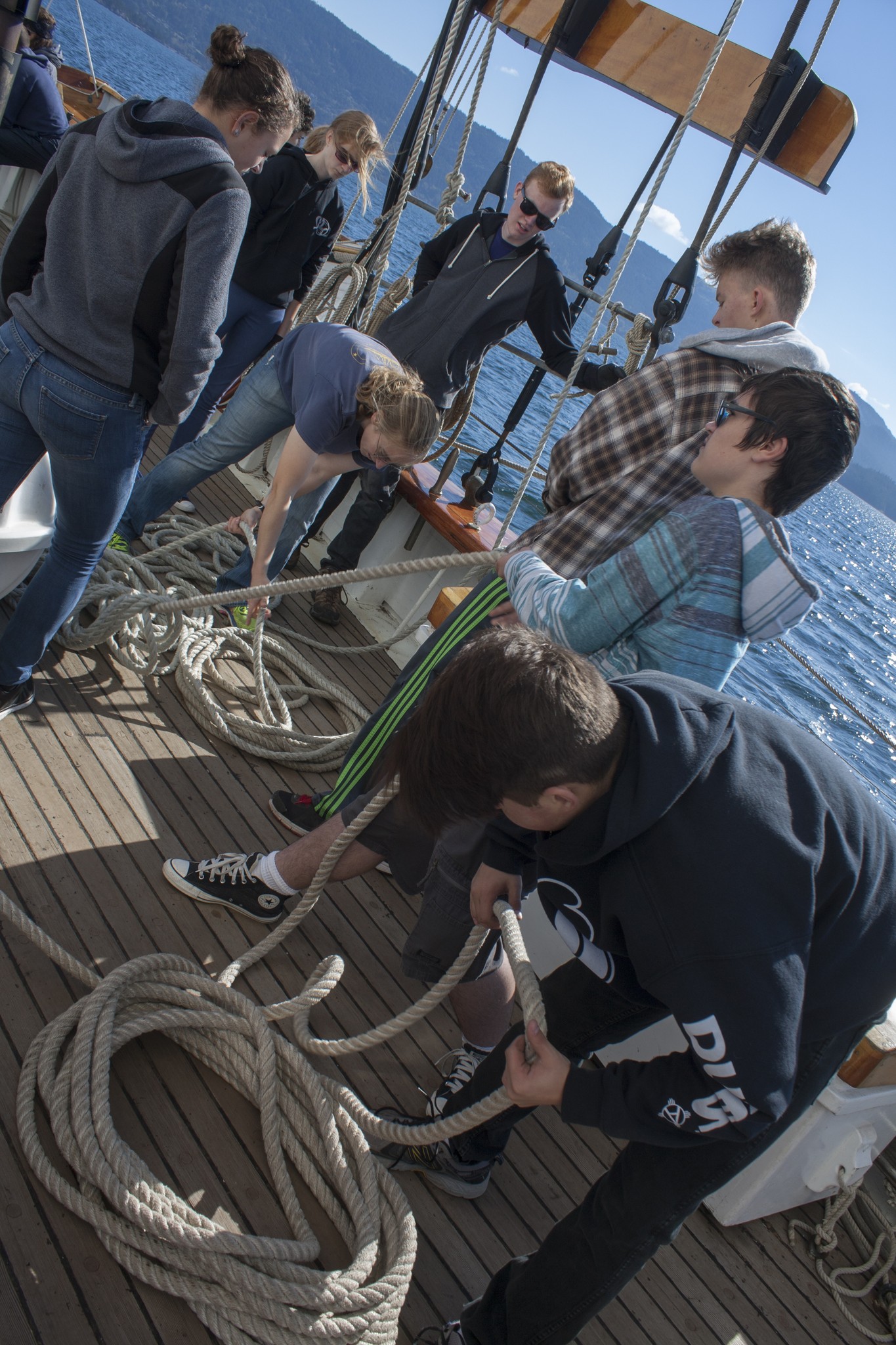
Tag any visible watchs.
[254,499,264,514]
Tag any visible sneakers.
[363,1109,491,1198]
[269,789,325,836]
[282,539,309,570]
[108,531,128,556]
[0,673,34,720]
[214,602,258,634]
[163,854,285,924]
[425,1038,503,1120]
[310,568,342,626]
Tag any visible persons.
[269,216,832,876]
[162,365,861,1112]
[23,6,63,85]
[113,320,439,631]
[136,111,385,513]
[356,630,896,1345]
[0,25,295,716]
[289,91,313,147]
[283,160,623,628]
[0,24,70,175]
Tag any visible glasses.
[333,136,363,172]
[520,185,559,232]
[374,427,406,472]
[714,399,778,431]
